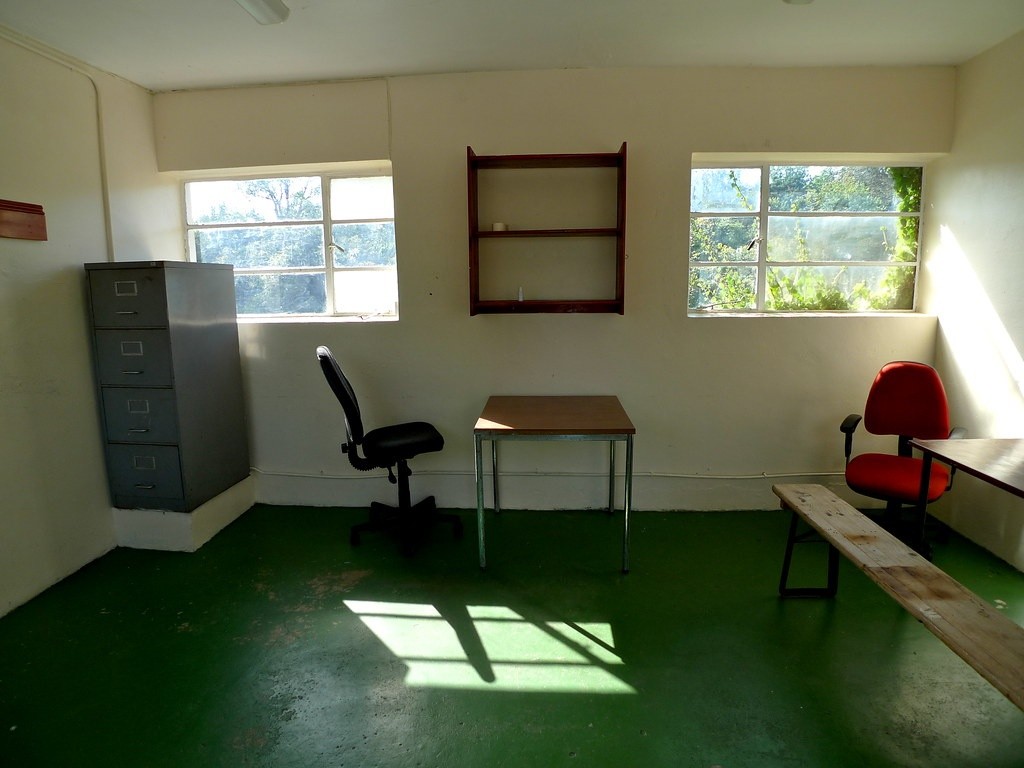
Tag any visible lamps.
[234,0,291,26]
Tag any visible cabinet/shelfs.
[83,260,251,511]
[462,140,630,316]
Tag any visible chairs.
[316,344,462,559]
[842,360,957,561]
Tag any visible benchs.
[770,484,1024,714]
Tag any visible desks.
[472,396,637,572]
[906,436,1024,551]
